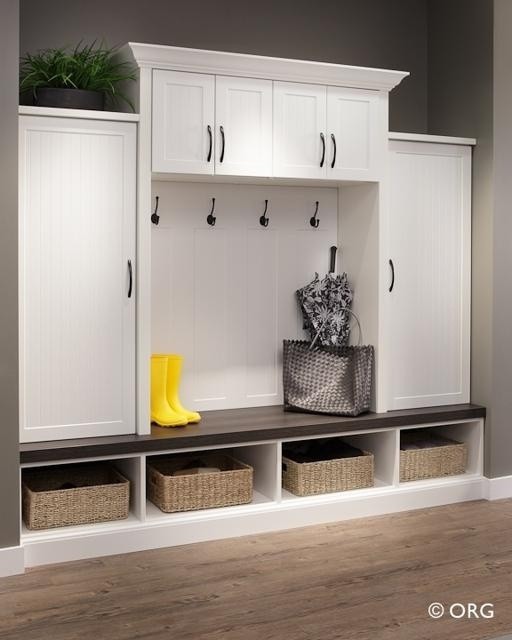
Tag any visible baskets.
[398,431,468,482]
[22,464,131,531]
[282,444,378,497]
[147,451,258,514]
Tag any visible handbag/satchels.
[281,309,376,417]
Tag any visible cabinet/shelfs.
[21,407,486,569]
[154,62,379,190]
[17,117,135,441]
[334,142,472,411]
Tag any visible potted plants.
[18,36,139,108]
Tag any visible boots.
[150,353,189,429]
[164,351,203,425]
